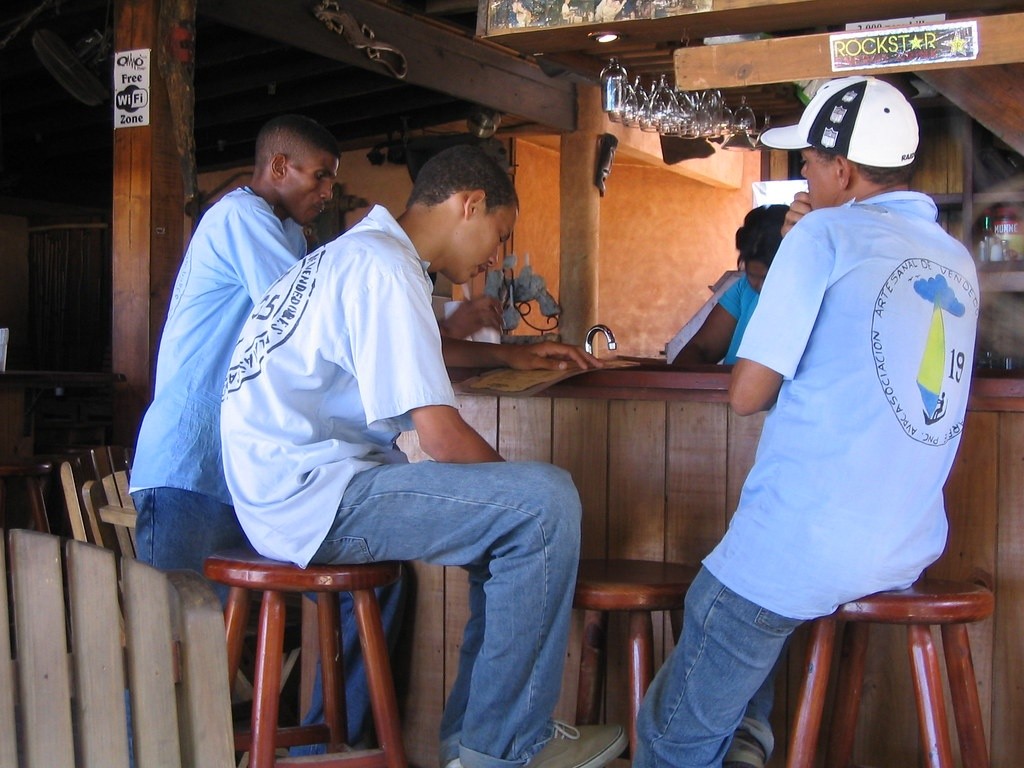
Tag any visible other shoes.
[722,729,765,768]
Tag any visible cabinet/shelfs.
[788,95,1024,380]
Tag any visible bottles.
[976,351,1017,370]
[978,207,1024,261]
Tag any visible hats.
[759,75,919,168]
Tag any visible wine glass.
[600,57,772,150]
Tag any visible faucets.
[584,324,617,355]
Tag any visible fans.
[30,23,122,108]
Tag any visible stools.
[205,544,410,768]
[0,458,73,661]
[786,576,996,768]
[571,557,701,768]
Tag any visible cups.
[443,301,471,340]
[471,326,501,344]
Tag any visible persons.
[630,78,981,768]
[121,115,406,768]
[223,142,630,768]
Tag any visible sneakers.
[525,721,630,768]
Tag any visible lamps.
[586,30,622,44]
[482,162,563,337]
[59,445,258,768]
[403,130,510,183]
[593,132,618,198]
